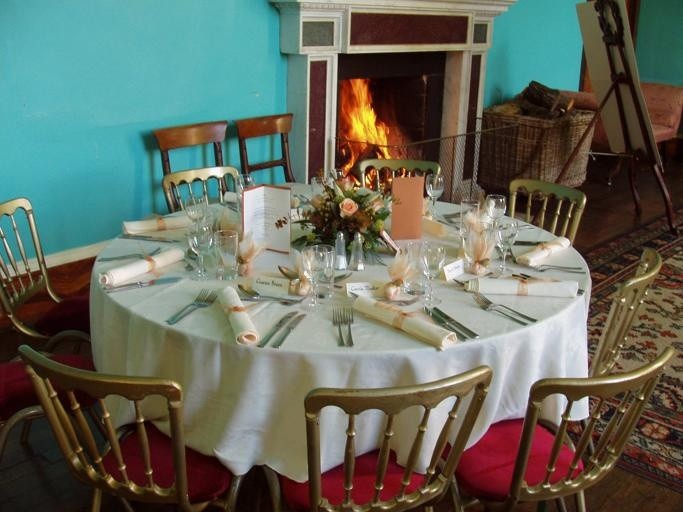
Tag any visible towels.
[223,191,240,203]
[121,216,189,235]
[218,284,258,347]
[421,217,446,238]
[464,276,580,299]
[352,295,456,351]
[517,237,568,267]
[99,245,186,288]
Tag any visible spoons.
[237,282,298,305]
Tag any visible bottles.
[347,233,367,271]
[334,231,346,270]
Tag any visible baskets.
[477,101,597,200]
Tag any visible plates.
[276,264,351,288]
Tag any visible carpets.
[564,205,680,492]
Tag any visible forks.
[475,293,538,326]
[340,309,357,347]
[330,306,343,347]
[165,289,217,325]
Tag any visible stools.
[2,355,102,465]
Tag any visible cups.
[309,243,334,301]
[308,165,344,213]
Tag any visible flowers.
[289,172,394,262]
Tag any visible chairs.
[443,344,675,510]
[162,166,242,215]
[638,81,683,168]
[559,89,627,190]
[18,345,248,512]
[587,245,663,378]
[259,364,494,511]
[152,121,232,211]
[234,112,298,192]
[1,198,95,363]
[508,177,587,250]
[358,158,442,201]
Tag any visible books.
[240,182,292,255]
[390,167,426,241]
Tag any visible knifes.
[433,305,481,339]
[258,312,296,348]
[275,314,306,349]
[424,306,466,340]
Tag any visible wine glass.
[99,174,255,295]
[402,171,587,304]
[299,246,327,313]
